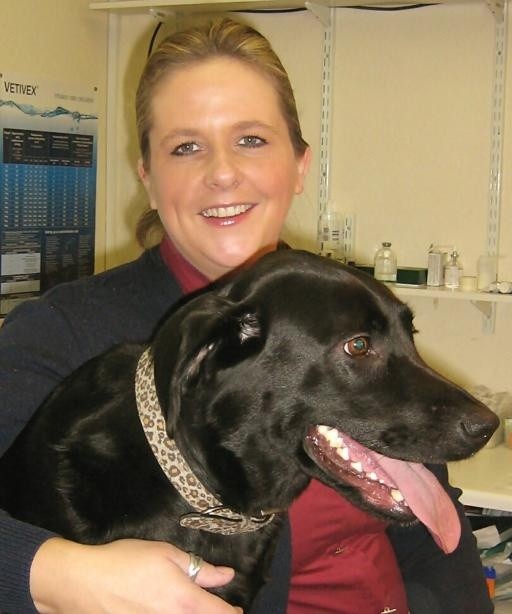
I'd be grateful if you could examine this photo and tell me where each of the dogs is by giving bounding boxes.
[0,250,500,614]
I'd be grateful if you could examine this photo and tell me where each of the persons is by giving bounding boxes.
[2,15,494,612]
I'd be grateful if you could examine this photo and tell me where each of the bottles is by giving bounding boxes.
[373,241,396,280]
[444,250,462,289]
[315,211,341,260]
[475,254,495,288]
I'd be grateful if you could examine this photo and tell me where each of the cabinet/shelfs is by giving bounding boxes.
[89,0,512,513]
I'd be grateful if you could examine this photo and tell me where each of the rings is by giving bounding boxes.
[185,549,204,582]
